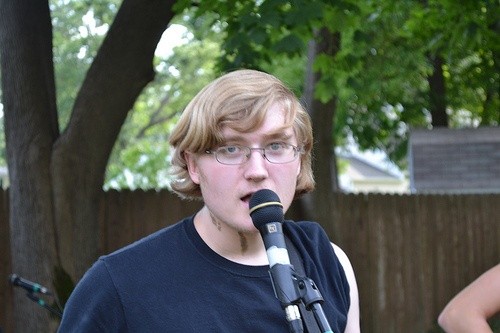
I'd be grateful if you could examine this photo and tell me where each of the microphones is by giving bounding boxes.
[9,274,51,295]
[249,189,304,333]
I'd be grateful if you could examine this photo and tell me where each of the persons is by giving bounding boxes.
[57,67,362,333]
[436,260,500,332]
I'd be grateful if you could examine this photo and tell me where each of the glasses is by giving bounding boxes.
[203,141,302,166]
